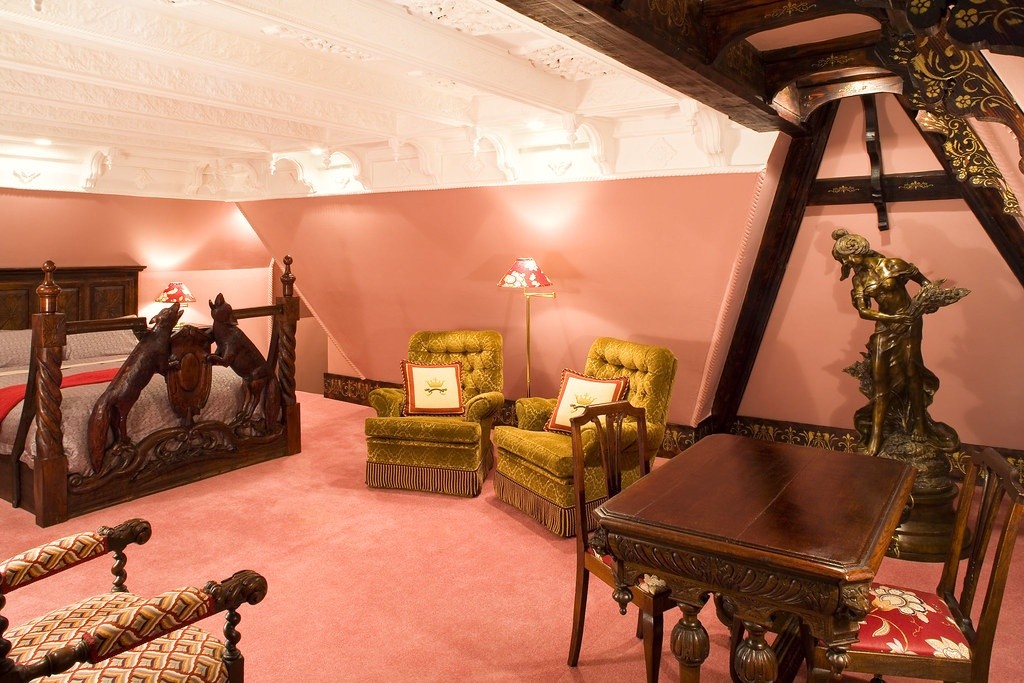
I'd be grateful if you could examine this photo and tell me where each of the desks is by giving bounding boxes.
[591,434,919,683]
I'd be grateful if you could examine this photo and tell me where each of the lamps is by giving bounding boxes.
[497,259,558,401]
[154,282,196,328]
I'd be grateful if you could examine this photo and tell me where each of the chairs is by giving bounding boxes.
[0,510,267,683]
[567,401,676,683]
[814,448,1024,683]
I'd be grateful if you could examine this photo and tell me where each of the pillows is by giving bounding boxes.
[399,357,464,417]
[544,368,628,438]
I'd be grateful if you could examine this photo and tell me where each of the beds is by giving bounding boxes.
[0,255,303,528]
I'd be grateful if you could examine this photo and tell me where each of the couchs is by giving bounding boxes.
[365,330,503,499]
[491,335,677,538]
